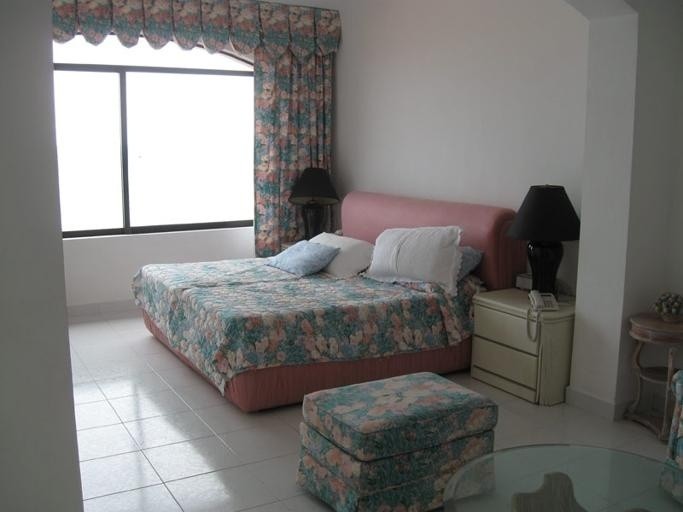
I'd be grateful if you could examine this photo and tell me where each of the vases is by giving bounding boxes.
[661,312,679,323]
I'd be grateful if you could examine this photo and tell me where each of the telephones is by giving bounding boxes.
[528,290,560,311]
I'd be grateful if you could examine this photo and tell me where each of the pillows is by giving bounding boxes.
[262,223,484,297]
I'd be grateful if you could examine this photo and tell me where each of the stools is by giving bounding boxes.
[625,313,681,443]
[297,371,500,511]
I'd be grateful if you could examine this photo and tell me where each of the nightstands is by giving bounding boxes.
[467,288,575,407]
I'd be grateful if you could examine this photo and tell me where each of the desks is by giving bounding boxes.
[443,443,682,510]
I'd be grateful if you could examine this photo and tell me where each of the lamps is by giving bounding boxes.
[286,167,340,241]
[503,183,580,299]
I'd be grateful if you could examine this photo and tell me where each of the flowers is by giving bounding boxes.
[652,291,682,314]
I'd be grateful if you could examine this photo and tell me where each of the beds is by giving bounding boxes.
[130,191,527,412]
